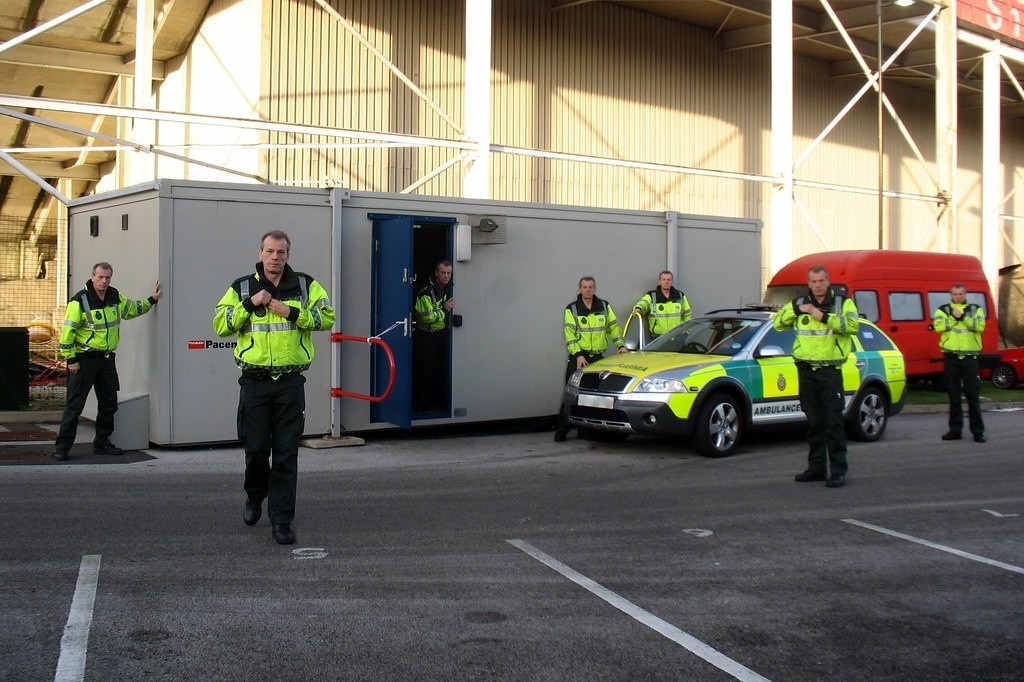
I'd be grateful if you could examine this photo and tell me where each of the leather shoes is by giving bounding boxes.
[973,434,986,442]
[942,431,962,440]
[795,470,826,482]
[825,475,846,488]
[272,525,295,544]
[94,446,123,455]
[54,447,69,461]
[243,498,262,525]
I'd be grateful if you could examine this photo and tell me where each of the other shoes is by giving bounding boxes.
[554,431,568,442]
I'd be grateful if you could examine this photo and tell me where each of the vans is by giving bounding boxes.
[764,252,1000,386]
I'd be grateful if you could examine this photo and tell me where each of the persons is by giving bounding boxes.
[212,230,336,545]
[773,266,859,487]
[554,277,627,442]
[413,260,456,411]
[934,284,987,443]
[53,262,162,461]
[632,271,694,343]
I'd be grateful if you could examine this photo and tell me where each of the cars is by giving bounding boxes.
[991,346,1024,390]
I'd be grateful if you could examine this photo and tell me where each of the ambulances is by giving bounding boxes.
[562,301,908,457]
